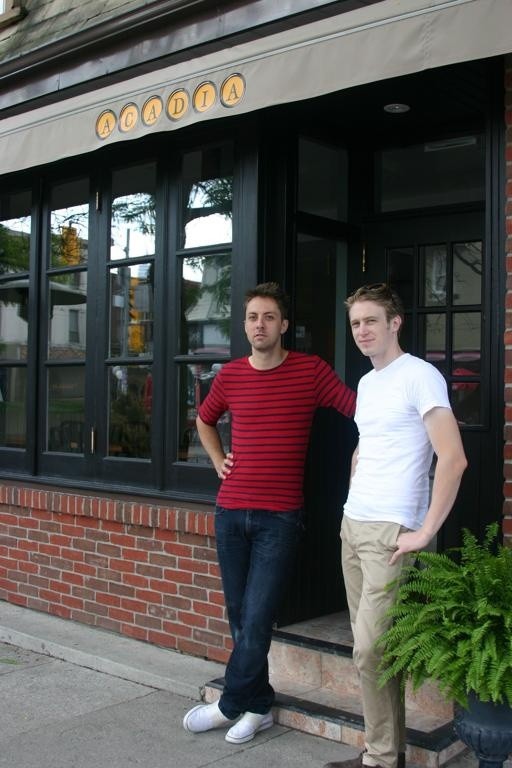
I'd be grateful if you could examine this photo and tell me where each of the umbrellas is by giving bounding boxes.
[323,752,381,767]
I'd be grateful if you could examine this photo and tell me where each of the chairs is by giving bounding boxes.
[224,708,274,744]
[183,700,233,734]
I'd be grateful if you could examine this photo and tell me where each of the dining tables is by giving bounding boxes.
[368,523,511,768]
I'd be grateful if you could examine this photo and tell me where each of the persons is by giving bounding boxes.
[180,280,356,745]
[324,282,470,766]
